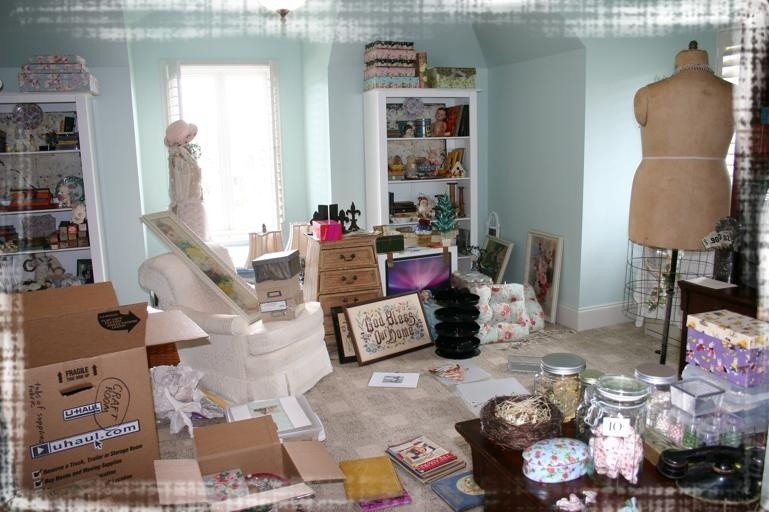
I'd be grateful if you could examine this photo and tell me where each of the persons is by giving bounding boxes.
[161,118,208,244]
[429,107,448,137]
[621,48,740,254]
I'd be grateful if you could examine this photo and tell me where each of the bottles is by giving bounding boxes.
[532,351,676,488]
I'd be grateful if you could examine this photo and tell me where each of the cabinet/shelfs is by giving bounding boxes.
[1,92,107,291]
[302,236,384,342]
[366,83,477,250]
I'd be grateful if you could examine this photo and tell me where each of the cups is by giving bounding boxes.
[671,378,725,415]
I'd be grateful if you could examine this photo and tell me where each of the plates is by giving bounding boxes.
[389,164,405,180]
[12,104,43,130]
[55,177,90,207]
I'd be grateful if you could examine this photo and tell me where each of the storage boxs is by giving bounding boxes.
[154,413,347,512]
[0,281,210,512]
[682,363,767,435]
[682,311,768,388]
[258,290,305,321]
[249,248,302,303]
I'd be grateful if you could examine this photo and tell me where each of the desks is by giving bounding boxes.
[453,417,767,511]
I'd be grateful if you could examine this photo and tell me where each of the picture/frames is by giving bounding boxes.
[478,236,515,283]
[141,212,258,318]
[523,231,564,326]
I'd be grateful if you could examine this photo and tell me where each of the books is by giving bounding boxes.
[336,452,404,504]
[356,484,416,510]
[428,470,487,511]
[446,147,465,173]
[385,432,467,487]
[447,105,471,137]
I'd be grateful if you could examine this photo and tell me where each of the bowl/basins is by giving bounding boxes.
[396,119,432,137]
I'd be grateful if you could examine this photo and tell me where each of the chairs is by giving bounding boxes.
[139,239,333,405]
